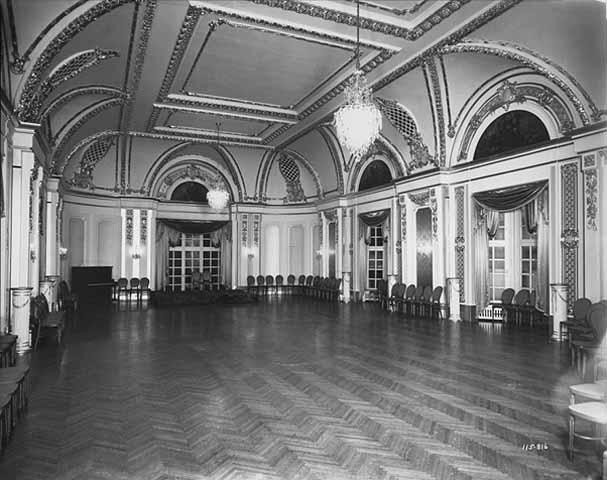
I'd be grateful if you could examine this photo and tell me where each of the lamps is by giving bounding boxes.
[206,121,231,211]
[331,0,383,162]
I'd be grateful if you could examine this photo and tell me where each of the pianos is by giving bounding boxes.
[71,267,115,307]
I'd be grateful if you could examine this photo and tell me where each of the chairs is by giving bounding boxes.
[34,281,79,353]
[492,288,536,329]
[247,274,341,303]
[118,278,151,302]
[378,279,444,320]
[0,336,31,450]
[559,298,607,480]
